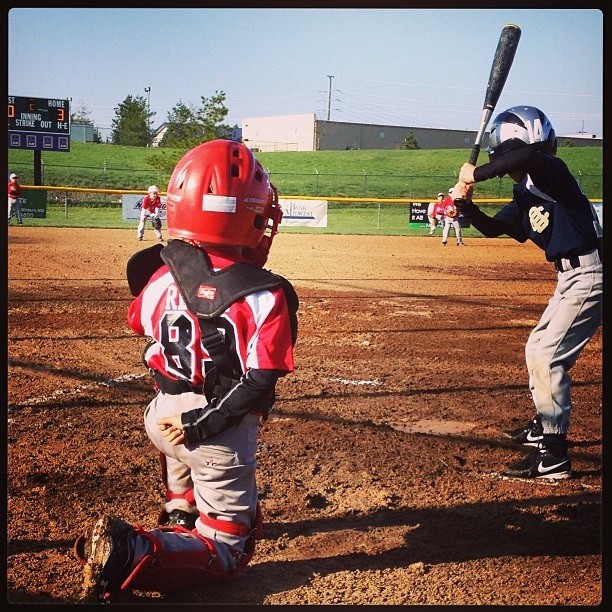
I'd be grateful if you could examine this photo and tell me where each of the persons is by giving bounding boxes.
[138,186,166,242]
[442,188,465,246]
[428,192,445,237]
[8,173,23,226]
[80,139,298,606]
[450,105,602,482]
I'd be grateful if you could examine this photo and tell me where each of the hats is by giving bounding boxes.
[10,174,20,179]
[448,188,454,193]
[437,193,444,197]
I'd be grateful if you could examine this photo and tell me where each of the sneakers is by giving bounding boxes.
[442,242,447,245]
[81,514,138,605]
[139,238,143,240]
[458,242,465,246]
[503,440,574,480]
[500,412,548,452]
[158,238,163,241]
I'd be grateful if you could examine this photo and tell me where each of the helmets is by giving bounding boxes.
[148,185,160,194]
[165,139,284,268]
[484,106,558,179]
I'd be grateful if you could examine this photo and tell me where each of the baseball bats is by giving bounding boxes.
[454,24,521,208]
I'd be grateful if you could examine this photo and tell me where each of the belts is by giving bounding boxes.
[553,257,580,273]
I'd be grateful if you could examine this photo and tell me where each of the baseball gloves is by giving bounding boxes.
[448,209,458,217]
[432,218,438,226]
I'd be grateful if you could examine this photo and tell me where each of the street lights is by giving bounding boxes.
[144,86,150,146]
